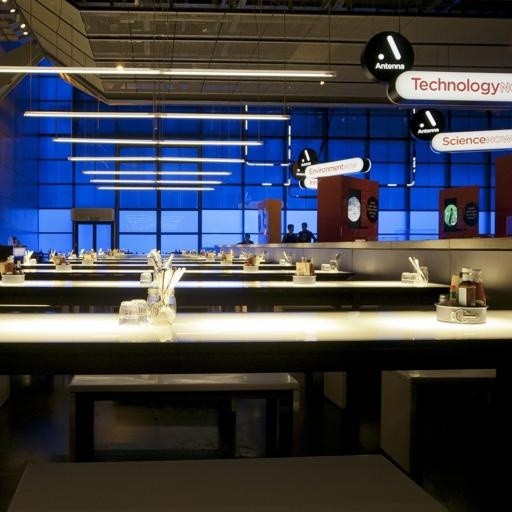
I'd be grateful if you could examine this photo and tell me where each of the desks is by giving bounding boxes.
[0,253,512,512]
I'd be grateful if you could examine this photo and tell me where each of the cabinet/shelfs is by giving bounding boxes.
[257,175,479,243]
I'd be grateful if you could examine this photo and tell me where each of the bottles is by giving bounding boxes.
[437,266,487,307]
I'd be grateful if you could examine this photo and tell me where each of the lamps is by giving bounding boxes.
[1,0,340,91]
[63,150,247,195]
[17,73,297,127]
[43,119,274,151]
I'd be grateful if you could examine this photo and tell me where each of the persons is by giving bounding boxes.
[295,222,317,243]
[242,233,254,244]
[279,223,298,243]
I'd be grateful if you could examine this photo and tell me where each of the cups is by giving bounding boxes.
[118,298,149,327]
